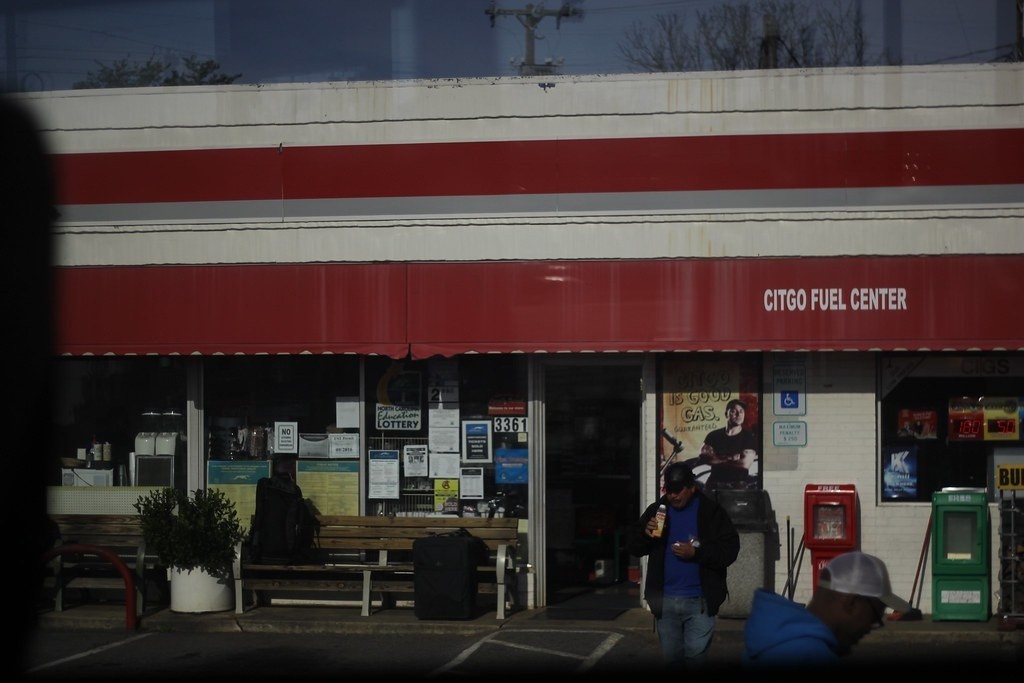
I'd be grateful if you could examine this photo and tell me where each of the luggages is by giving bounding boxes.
[411,534,477,620]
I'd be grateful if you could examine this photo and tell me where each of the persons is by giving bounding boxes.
[627,462,741,664]
[700,400,754,490]
[741,553,911,661]
[239,460,302,566]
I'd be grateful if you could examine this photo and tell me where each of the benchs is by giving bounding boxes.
[232,514,520,620]
[43,514,168,615]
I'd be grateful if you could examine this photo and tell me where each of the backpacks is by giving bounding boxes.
[249,473,304,560]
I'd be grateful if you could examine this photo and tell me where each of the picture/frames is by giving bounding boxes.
[462,420,492,463]
[368,449,399,499]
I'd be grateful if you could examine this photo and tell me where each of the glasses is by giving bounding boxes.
[666,487,682,494]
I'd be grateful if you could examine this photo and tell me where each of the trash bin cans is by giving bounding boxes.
[707,488,773,620]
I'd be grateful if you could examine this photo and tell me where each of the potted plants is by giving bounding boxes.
[129,487,248,613]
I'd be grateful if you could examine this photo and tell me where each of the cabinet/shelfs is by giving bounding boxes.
[931,487,989,621]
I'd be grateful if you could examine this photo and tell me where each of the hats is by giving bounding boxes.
[727,399,748,409]
[663,463,695,486]
[817,552,912,615]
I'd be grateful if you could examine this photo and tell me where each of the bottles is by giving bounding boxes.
[90,435,102,461]
[651,505,665,537]
[103,442,112,461]
